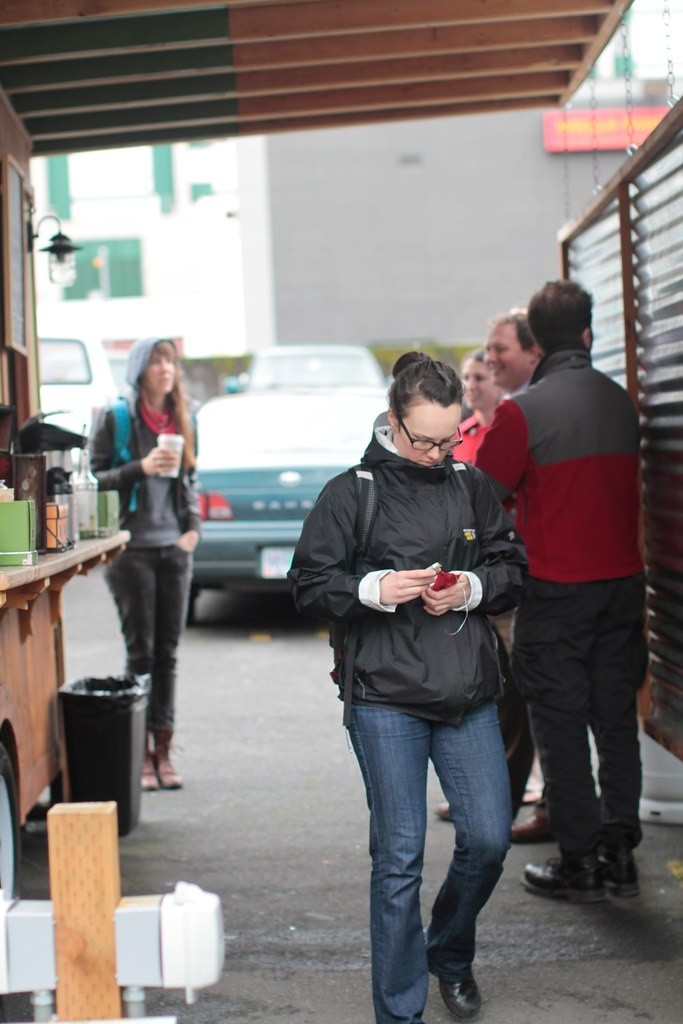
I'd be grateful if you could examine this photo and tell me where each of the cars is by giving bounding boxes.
[224,346,394,395]
[186,387,391,625]
[38,327,117,472]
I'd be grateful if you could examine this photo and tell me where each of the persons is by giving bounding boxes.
[91,337,201,789]
[435,310,555,844]
[476,280,646,903]
[286,351,530,1024]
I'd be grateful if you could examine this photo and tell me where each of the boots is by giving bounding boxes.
[154,727,183,789]
[140,730,159,792]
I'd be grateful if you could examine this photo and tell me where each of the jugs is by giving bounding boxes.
[19,423,89,470]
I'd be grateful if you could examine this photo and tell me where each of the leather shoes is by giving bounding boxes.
[426,953,481,1018]
[599,855,639,898]
[511,815,554,845]
[435,803,451,822]
[520,854,608,902]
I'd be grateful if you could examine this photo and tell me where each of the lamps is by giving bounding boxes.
[26,214,83,285]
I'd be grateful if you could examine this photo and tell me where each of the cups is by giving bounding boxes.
[156,434,184,478]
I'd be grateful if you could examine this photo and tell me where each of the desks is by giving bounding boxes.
[0,529,130,643]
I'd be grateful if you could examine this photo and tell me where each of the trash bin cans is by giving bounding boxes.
[56,674,152,837]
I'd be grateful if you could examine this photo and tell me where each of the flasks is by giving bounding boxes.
[46,467,74,544]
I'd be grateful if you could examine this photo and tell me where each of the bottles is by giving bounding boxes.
[71,449,99,539]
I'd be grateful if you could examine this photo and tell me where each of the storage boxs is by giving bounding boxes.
[0,491,119,566]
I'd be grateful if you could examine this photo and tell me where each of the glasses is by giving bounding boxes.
[396,412,462,450]
